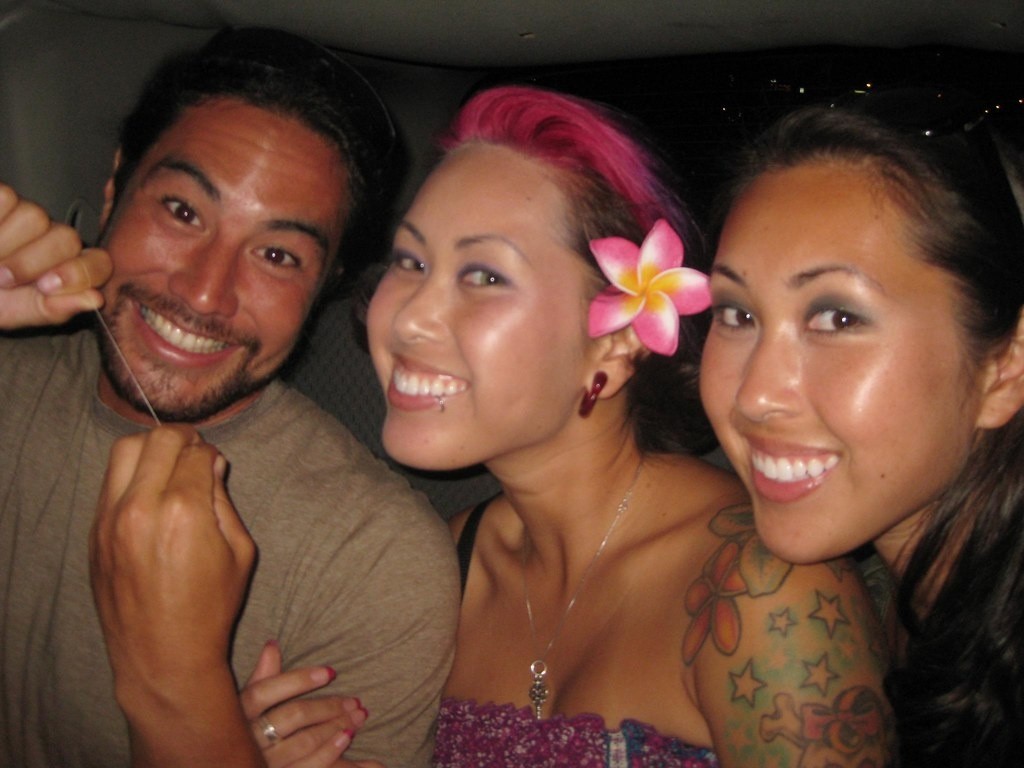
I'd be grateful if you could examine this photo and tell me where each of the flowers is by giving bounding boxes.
[587,218,712,356]
[681,501,854,667]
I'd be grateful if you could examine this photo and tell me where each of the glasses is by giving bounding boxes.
[719,66,1024,137]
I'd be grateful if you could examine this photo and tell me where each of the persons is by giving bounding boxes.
[232,88,897,767]
[0,25,461,767]
[693,101,1024,768]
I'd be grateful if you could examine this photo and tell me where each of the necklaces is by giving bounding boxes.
[521,448,645,722]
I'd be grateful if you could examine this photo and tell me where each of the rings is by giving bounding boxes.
[257,713,283,746]
